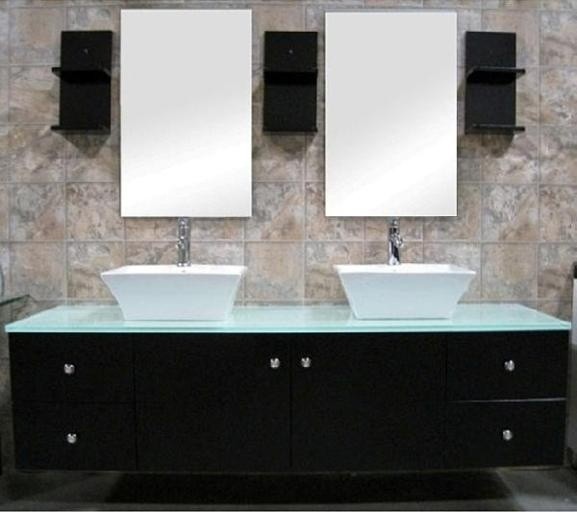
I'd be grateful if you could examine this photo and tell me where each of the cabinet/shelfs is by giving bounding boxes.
[260,30,319,133]
[463,30,525,135]
[8,333,568,470]
[50,30,113,135]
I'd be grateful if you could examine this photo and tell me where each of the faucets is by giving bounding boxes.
[175,218,190,265]
[387,217,402,266]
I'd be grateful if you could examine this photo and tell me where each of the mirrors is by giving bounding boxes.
[324,12,458,219]
[120,9,252,220]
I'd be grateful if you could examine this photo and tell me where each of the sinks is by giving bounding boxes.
[334,264,477,320]
[101,265,242,320]
